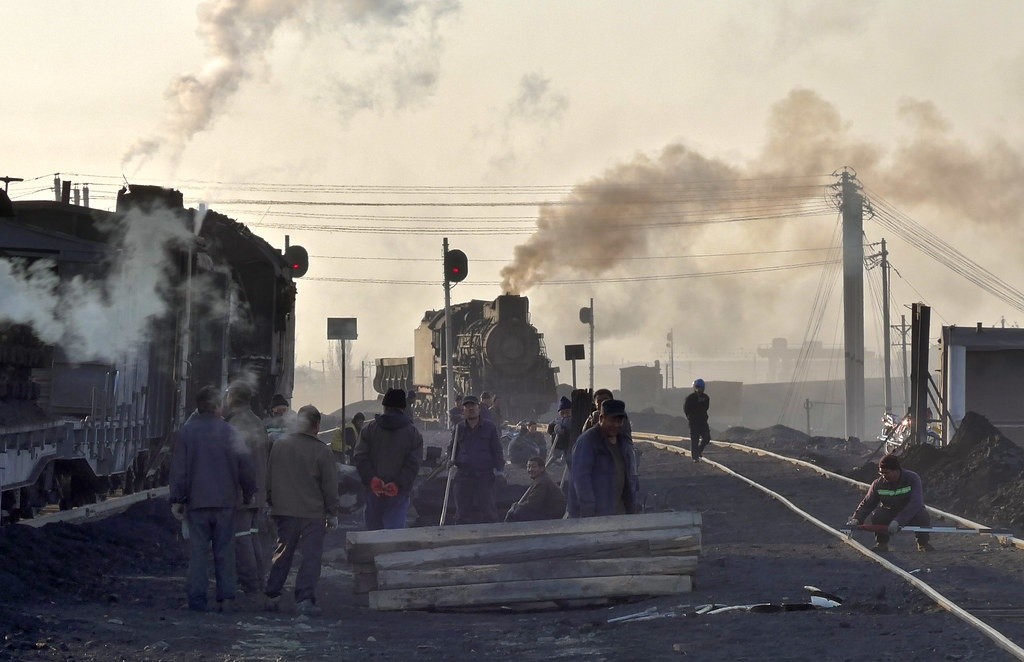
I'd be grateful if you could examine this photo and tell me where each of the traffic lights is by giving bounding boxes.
[446,250,468,283]
[285,245,308,278]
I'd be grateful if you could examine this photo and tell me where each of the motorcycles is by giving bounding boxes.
[876,407,941,456]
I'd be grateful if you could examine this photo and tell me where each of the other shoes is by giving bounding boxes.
[191,602,233,613]
[248,579,268,592]
[693,446,702,463]
[267,596,322,616]
[871,542,935,552]
[177,604,189,610]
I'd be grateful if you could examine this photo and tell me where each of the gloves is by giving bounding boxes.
[845,519,899,534]
[370,479,398,497]
[327,518,338,529]
[249,495,256,504]
[266,506,272,519]
[554,424,561,433]
[171,503,186,520]
[447,460,456,469]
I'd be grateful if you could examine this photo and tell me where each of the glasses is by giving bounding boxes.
[878,472,889,476]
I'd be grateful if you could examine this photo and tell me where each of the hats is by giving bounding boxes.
[462,396,479,405]
[558,396,572,412]
[879,455,901,470]
[480,392,491,398]
[382,388,406,409]
[408,391,416,397]
[601,399,627,418]
[455,396,462,401]
[225,381,250,403]
[270,394,289,408]
[520,425,529,435]
[354,413,365,421]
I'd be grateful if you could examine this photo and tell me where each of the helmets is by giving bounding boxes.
[692,378,705,387]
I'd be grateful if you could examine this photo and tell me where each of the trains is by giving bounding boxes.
[372,294,559,424]
[0,179,296,524]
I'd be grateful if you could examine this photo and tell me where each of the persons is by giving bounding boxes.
[169,387,258,616]
[354,388,423,529]
[403,390,416,422]
[685,378,711,463]
[447,386,638,526]
[330,411,364,466]
[847,455,933,553]
[225,380,270,601]
[264,394,297,450]
[262,405,340,617]
